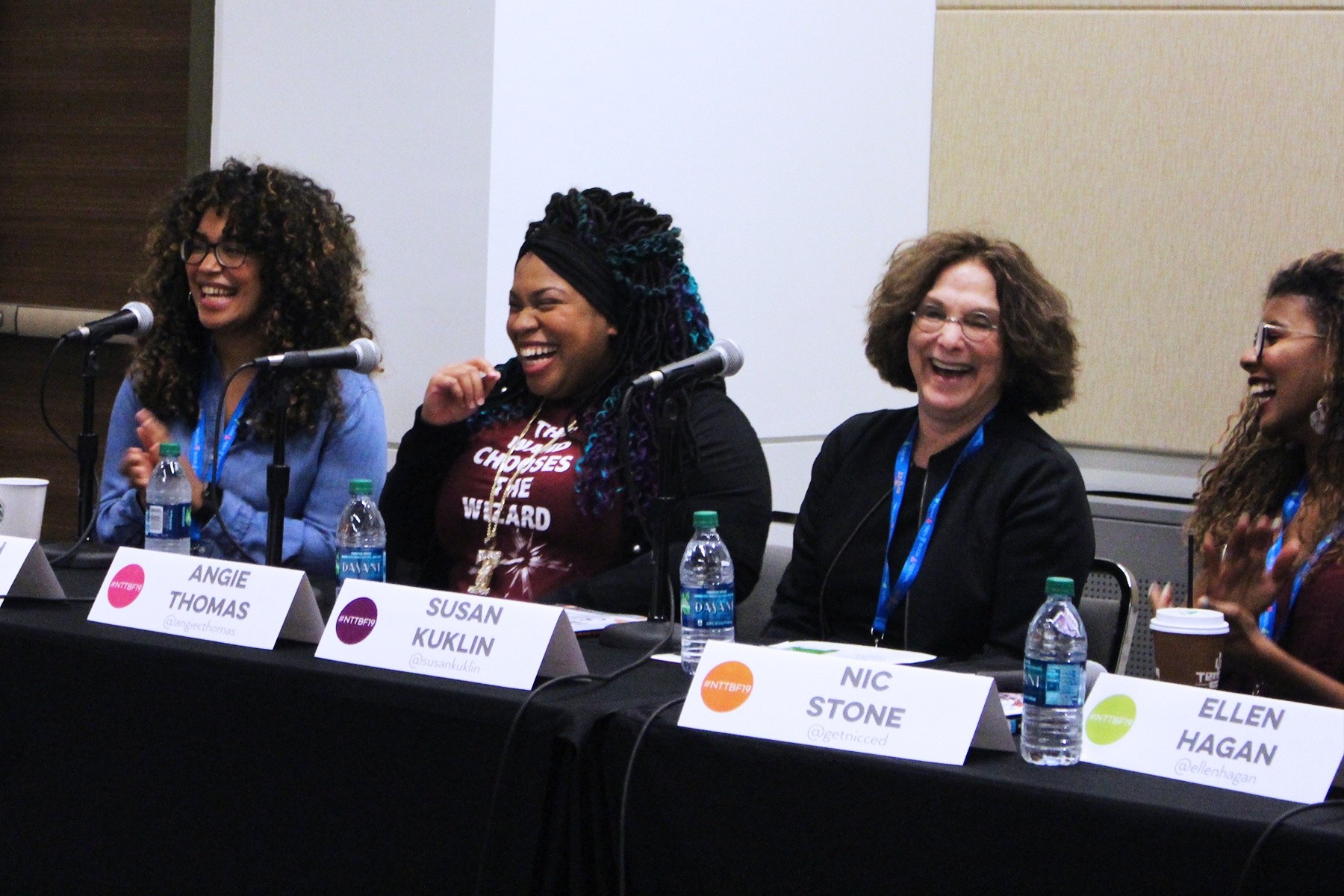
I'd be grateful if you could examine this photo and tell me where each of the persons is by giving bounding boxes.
[1145,248,1344,721]
[761,231,1097,663]
[93,163,391,586]
[374,187,772,631]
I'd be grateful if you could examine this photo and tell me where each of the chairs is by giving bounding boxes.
[736,509,800,646]
[1078,558,1139,676]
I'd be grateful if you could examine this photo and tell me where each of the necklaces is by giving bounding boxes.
[467,402,582,597]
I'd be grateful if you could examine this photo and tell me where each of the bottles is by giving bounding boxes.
[336,479,387,602]
[145,443,192,558]
[680,511,735,676]
[1020,577,1089,767]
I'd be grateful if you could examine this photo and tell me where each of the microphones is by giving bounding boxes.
[251,338,383,374]
[61,302,154,341]
[632,338,745,392]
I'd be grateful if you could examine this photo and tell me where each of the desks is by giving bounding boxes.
[0,554,1344,896]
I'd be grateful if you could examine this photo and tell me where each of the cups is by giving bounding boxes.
[0,477,50,544]
[1149,607,1231,689]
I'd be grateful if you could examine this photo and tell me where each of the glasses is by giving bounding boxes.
[910,306,1005,343]
[1253,320,1325,361]
[182,233,264,268]
